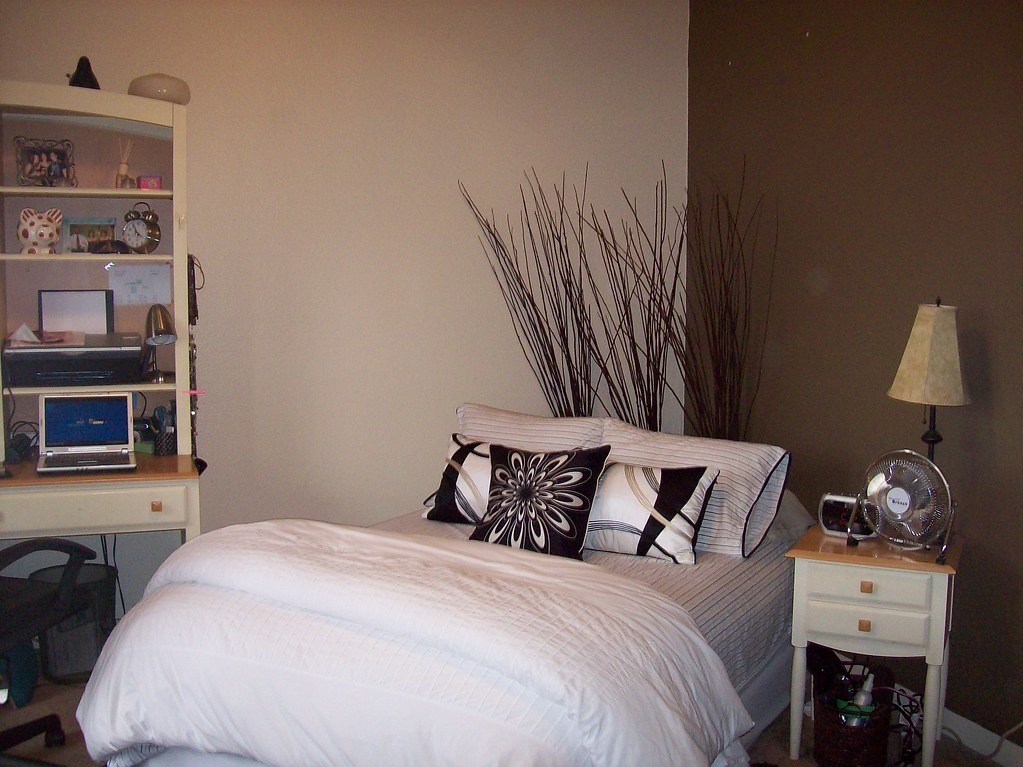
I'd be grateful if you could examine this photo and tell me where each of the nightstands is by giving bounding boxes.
[786,530,960,767]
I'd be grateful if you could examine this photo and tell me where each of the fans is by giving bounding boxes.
[848,447,958,564]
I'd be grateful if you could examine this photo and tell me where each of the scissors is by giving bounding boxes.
[153,406,168,433]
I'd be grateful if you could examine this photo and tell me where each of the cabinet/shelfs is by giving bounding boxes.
[0,76,199,542]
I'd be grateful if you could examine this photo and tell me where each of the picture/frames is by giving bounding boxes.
[61,216,117,255]
[14,136,79,188]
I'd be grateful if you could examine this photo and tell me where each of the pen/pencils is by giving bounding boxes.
[149,424,156,434]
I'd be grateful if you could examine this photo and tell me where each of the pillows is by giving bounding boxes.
[469,445,611,562]
[456,405,601,451]
[602,416,791,558]
[421,432,497,526]
[584,461,721,565]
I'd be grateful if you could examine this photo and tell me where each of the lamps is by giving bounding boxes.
[144,304,179,381]
[884,294,972,543]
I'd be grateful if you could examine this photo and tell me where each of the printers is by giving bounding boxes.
[3,332,143,388]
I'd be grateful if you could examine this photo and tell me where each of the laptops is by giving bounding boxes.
[36,392,137,473]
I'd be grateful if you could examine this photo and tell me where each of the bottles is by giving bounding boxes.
[845,673,874,727]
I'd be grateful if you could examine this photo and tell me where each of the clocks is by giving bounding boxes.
[121,202,161,255]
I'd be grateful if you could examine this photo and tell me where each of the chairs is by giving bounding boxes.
[0,537,98,767]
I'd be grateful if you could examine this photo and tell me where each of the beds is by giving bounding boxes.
[76,404,819,765]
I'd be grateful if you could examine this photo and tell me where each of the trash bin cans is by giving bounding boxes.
[812,660,894,767]
[27,563,119,684]
